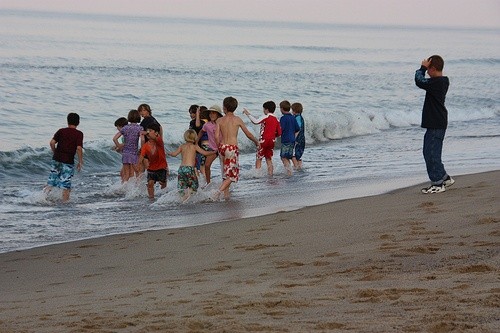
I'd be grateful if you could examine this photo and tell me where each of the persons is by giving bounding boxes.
[188,104,207,175]
[168,129,217,201]
[215,96,260,203]
[138,124,168,199]
[45,113,84,203]
[291,102,306,169]
[242,101,282,180]
[279,100,300,178]
[415,54,456,194]
[138,104,162,170]
[114,110,145,183]
[188,104,223,189]
[114,117,129,131]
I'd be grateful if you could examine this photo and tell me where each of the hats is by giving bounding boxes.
[206,104,223,117]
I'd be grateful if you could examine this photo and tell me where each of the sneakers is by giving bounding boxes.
[443,175,455,187]
[421,182,445,194]
